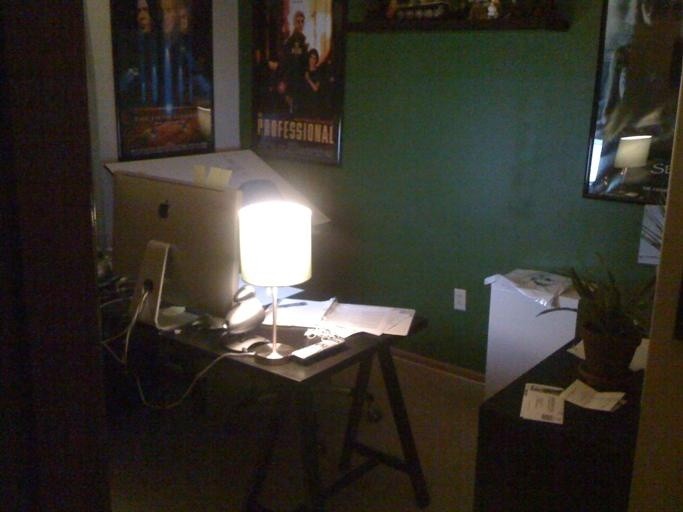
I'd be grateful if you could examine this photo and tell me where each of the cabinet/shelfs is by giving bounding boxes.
[475,331,648,511]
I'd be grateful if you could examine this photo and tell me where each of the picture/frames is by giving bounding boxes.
[254,2,344,164]
[362,0,573,30]
[108,0,213,161]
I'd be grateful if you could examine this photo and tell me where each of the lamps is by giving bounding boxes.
[613,133,652,197]
[238,201,312,363]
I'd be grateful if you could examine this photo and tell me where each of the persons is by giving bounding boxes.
[600,0,681,161]
[252,9,329,116]
[117,1,212,100]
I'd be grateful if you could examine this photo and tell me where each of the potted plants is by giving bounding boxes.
[533,250,656,383]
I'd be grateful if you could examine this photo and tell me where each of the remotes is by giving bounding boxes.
[291,339,346,364]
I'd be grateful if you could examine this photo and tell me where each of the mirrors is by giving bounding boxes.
[583,0,683,207]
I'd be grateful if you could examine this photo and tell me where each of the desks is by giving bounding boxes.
[101,286,429,512]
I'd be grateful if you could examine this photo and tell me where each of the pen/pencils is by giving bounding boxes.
[281,303,308,309]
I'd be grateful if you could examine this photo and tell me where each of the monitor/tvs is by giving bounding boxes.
[589,138,603,186]
[111,170,243,332]
